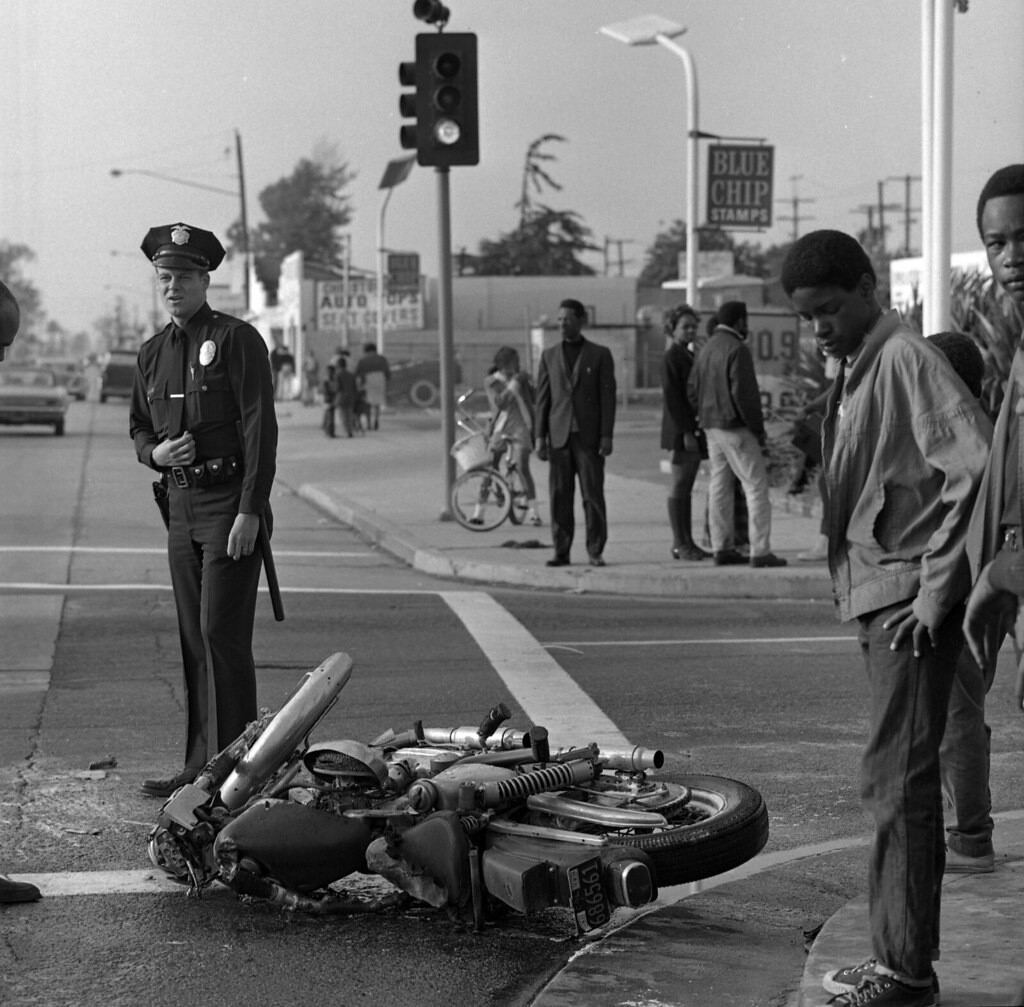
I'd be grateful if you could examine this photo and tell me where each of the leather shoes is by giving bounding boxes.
[139,766,201,797]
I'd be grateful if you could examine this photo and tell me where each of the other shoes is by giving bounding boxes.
[748,551,788,568]
[0,872,40,903]
[547,554,570,567]
[798,541,830,560]
[714,549,751,565]
[588,552,607,567]
[943,844,998,872]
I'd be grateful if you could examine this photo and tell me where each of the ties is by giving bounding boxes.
[168,325,186,443]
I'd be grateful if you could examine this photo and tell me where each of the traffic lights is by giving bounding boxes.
[400,31,482,167]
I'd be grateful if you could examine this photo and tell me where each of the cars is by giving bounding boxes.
[0,364,69,435]
[50,359,90,400]
[98,348,140,402]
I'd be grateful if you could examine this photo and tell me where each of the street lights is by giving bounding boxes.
[378,158,416,353]
[111,167,249,322]
[602,11,700,312]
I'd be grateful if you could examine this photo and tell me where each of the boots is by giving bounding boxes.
[665,493,714,561]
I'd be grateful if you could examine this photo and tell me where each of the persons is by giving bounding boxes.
[660,303,714,560]
[685,302,787,569]
[964,164,1024,712]
[279,344,297,399]
[303,349,319,406]
[0,284,43,904]
[332,357,364,437]
[356,343,390,429]
[703,317,750,551]
[270,341,281,398]
[321,364,335,437]
[780,227,994,1007]
[129,222,278,798]
[536,301,617,567]
[469,345,541,526]
[925,332,993,872]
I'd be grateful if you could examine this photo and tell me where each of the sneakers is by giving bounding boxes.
[822,953,941,1007]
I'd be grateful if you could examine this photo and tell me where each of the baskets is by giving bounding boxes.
[450,432,493,472]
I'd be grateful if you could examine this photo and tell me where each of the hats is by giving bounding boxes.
[141,223,225,275]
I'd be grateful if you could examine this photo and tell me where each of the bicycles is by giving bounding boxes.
[452,388,529,531]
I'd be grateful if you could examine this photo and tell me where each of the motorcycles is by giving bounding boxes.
[148,651,769,945]
